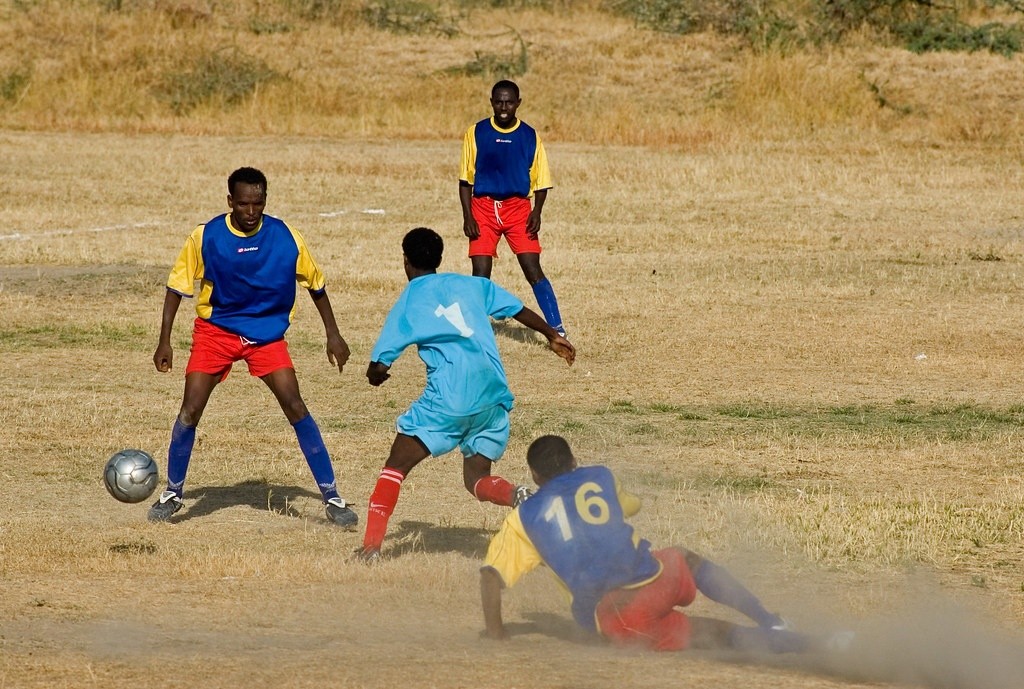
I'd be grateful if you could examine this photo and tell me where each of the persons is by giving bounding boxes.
[147,167,359,527]
[352,227,576,563]
[478,436,857,656]
[458,80,568,340]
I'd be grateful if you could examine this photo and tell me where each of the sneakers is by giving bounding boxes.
[347,547,385,571]
[511,485,539,509]
[145,490,185,526]
[322,497,360,527]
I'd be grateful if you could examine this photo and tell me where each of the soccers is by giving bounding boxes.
[105,450,158,502]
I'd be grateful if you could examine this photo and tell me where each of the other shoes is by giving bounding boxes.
[767,615,809,651]
[552,324,566,338]
